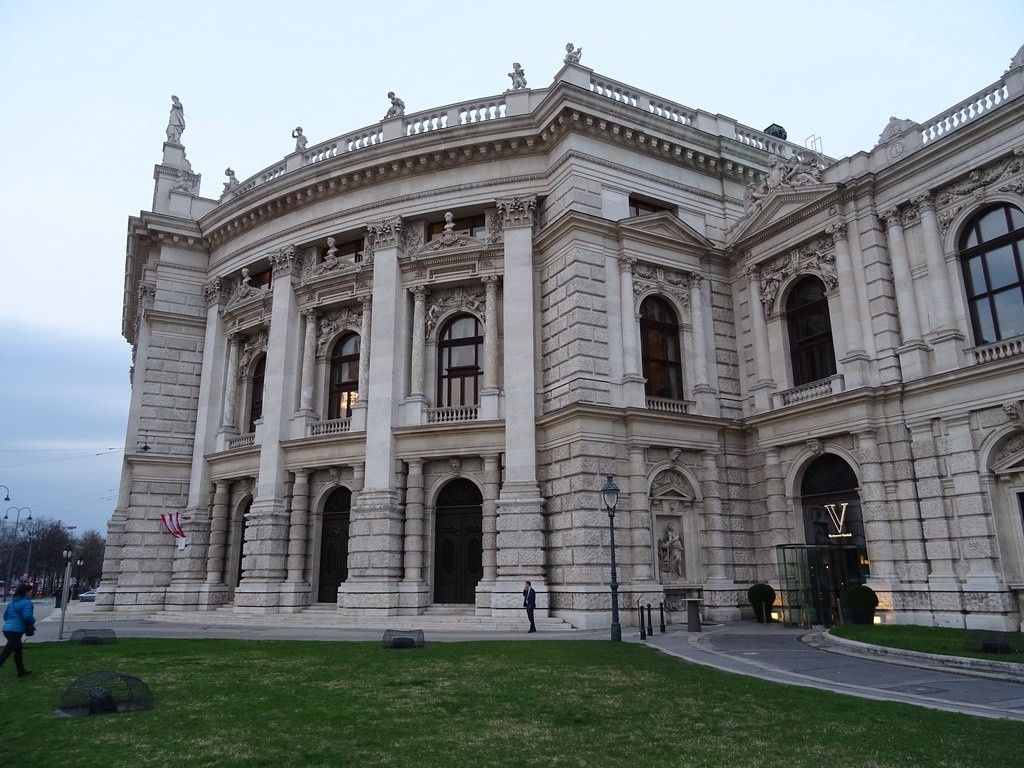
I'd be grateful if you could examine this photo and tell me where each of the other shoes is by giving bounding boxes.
[18,669,31,677]
[528,628,536,633]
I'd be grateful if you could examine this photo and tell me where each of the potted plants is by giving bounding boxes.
[843,585,879,625]
[748,583,776,623]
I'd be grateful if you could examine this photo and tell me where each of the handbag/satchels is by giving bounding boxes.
[26,621,34,638]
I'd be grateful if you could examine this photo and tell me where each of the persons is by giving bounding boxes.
[743,155,821,217]
[759,251,839,317]
[672,448,681,464]
[806,439,824,454]
[444,212,456,231]
[563,43,582,63]
[315,314,363,357]
[384,92,405,118]
[0,584,36,677]
[327,237,339,255]
[292,127,308,152]
[329,466,340,483]
[240,343,253,368]
[241,479,253,493]
[222,167,239,193]
[425,296,485,340]
[969,170,983,183]
[523,581,536,633]
[173,169,190,191]
[449,458,462,475]
[1002,402,1024,427]
[166,95,185,145]
[242,268,252,284]
[665,523,683,577]
[54,584,92,608]
[508,63,526,89]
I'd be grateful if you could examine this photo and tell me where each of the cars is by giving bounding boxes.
[78,590,97,602]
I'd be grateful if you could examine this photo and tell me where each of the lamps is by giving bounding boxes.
[393,638,416,648]
[82,637,103,645]
[89,687,118,715]
[142,445,151,450]
[982,639,1013,654]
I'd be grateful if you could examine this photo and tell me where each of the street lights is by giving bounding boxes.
[75,556,84,586]
[600,472,622,642]
[2,506,32,602]
[57,543,72,590]
